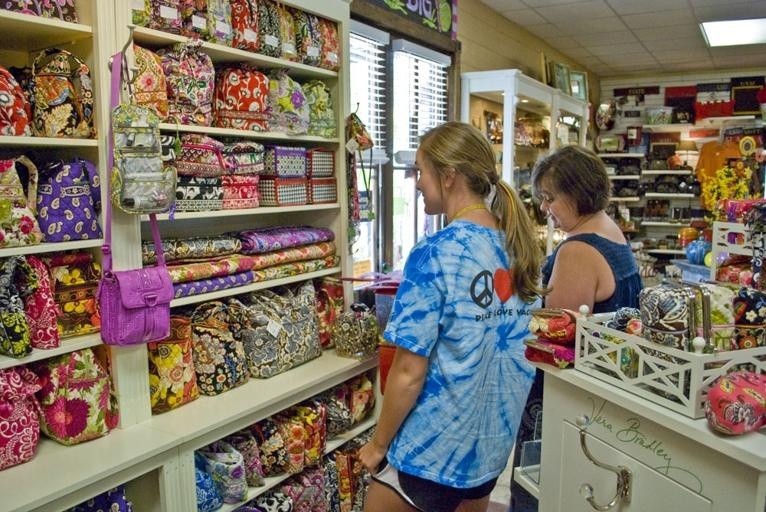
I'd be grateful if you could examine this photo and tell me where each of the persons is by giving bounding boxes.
[352,122,553,512]
[509,146,643,512]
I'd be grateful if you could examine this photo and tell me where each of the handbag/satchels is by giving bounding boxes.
[314,278,345,353]
[38,348,119,448]
[247,277,322,378]
[149,316,199,414]
[184,301,249,396]
[0,363,44,470]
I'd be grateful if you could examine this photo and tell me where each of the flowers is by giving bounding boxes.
[697,163,761,230]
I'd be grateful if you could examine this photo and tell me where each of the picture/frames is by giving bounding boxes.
[552,61,573,96]
[541,53,551,84]
[570,70,589,101]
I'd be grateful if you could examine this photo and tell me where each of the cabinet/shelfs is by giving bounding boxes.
[0,0,381,511]
[461,69,592,256]
[596,81,765,277]
[514,355,766,512]
